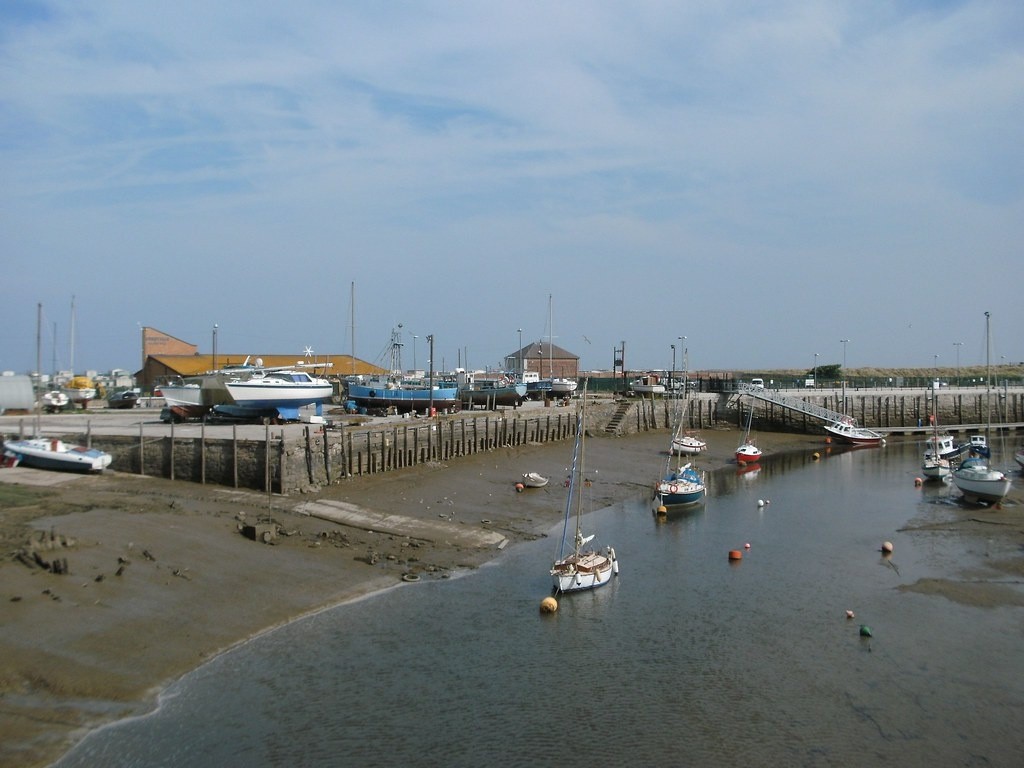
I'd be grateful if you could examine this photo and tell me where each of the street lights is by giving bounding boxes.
[841,339,850,386]
[952,343,964,387]
[814,353,820,389]
[934,354,939,381]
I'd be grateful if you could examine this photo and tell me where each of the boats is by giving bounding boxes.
[921,434,990,481]
[824,414,890,446]
[630,377,700,393]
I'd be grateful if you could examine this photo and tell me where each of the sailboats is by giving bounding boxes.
[657,344,706,513]
[667,336,706,455]
[5,302,115,471]
[0,282,577,425]
[552,377,614,595]
[950,310,1015,500]
[735,383,763,465]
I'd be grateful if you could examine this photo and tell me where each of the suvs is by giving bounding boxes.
[751,378,764,390]
[805,374,814,388]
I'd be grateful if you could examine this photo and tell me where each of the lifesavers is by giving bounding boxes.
[656,482,659,491]
[670,485,678,494]
